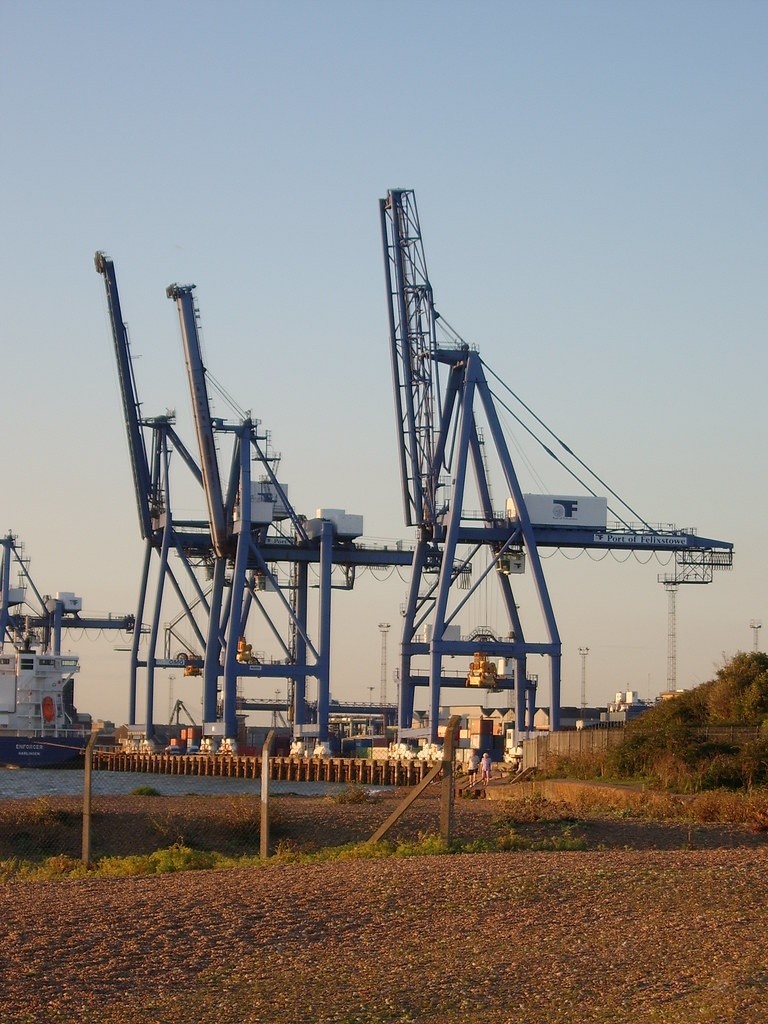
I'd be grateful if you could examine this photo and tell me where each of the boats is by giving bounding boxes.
[0,615,97,770]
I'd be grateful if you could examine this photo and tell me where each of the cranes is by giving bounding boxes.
[0,529,152,655]
[379,186,736,760]
[94,250,474,757]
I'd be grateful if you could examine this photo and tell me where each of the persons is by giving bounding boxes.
[466,750,480,788]
[480,753,492,786]
